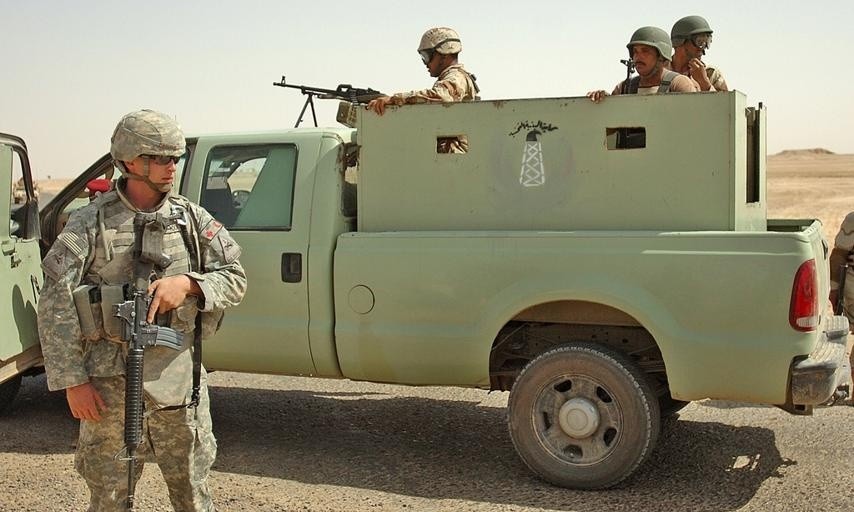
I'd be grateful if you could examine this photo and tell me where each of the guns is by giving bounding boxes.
[111,212,184,508]
[273,75,399,127]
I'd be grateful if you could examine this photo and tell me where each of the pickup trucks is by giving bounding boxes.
[0,90,847,493]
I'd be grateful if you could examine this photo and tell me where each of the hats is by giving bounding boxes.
[417,27,462,56]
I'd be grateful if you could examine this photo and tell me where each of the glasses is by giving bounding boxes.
[138,154,181,165]
[690,32,712,49]
[419,49,435,65]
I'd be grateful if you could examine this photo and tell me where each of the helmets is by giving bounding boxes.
[109,108,187,164]
[670,15,713,48]
[626,26,673,62]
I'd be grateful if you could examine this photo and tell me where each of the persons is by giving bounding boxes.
[662,15,729,91]
[829,211,854,408]
[13,174,40,206]
[37,109,247,511]
[364,28,480,153]
[586,26,697,147]
